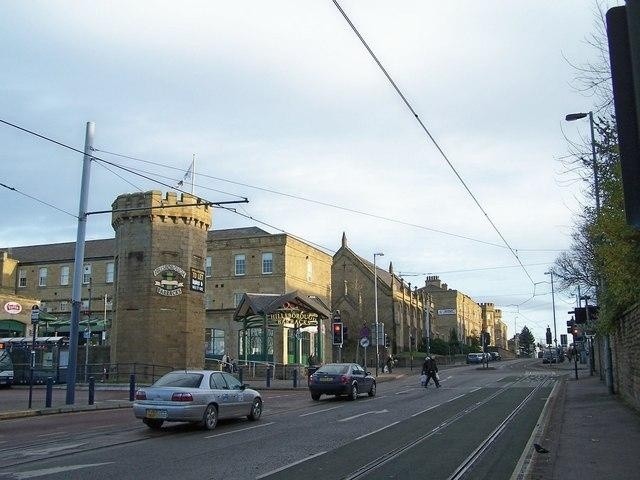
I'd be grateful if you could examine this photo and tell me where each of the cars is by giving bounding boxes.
[308,362,376,400]
[466,352,501,363]
[132,367,264,434]
[538,347,568,363]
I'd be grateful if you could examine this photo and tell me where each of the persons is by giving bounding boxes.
[420,353,442,388]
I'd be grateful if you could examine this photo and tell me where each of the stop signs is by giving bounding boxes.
[333,314,341,322]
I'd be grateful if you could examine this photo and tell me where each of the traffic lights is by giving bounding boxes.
[331,322,343,345]
[567,321,582,338]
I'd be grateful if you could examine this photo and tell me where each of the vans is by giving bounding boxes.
[0,349,14,388]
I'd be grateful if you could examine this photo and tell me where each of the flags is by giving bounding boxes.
[175,160,193,190]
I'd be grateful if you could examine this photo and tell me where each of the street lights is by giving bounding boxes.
[374,252,385,377]
[544,271,559,364]
